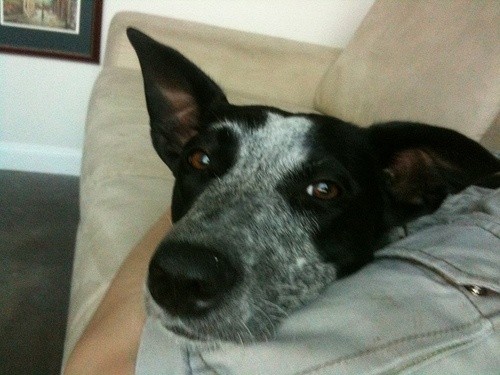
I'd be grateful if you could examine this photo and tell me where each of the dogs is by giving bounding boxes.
[126,26,500,346]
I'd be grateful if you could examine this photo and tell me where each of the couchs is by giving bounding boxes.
[62,0,500,375]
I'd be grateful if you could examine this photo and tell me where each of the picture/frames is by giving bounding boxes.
[0,0,103,66]
